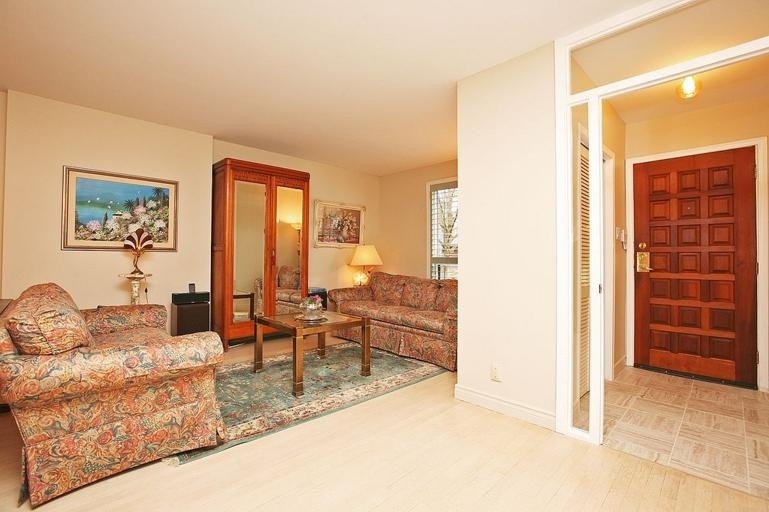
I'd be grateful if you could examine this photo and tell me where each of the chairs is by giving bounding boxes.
[0,301,224,510]
[254,264,301,313]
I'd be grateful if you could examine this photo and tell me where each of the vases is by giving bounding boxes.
[300,303,323,316]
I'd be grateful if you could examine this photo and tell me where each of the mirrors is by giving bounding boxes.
[233,180,264,323]
[276,185,303,314]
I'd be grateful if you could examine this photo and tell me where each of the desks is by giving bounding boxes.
[119,274,152,305]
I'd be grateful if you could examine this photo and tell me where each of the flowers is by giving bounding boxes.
[304,295,324,305]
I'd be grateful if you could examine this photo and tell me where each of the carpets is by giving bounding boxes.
[161,342,448,467]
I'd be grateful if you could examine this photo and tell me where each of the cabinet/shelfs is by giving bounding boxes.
[211,158,308,353]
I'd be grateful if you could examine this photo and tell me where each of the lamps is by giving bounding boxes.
[290,223,300,265]
[123,228,153,275]
[350,246,383,286]
[675,77,702,99]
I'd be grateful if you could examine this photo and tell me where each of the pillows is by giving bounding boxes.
[4,283,95,356]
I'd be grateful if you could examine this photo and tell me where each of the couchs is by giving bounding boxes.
[328,272,457,372]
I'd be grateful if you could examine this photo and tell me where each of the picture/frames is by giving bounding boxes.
[61,165,179,252]
[313,200,366,249]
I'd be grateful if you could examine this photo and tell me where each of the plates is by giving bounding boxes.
[297,319,329,325]
[294,314,326,321]
